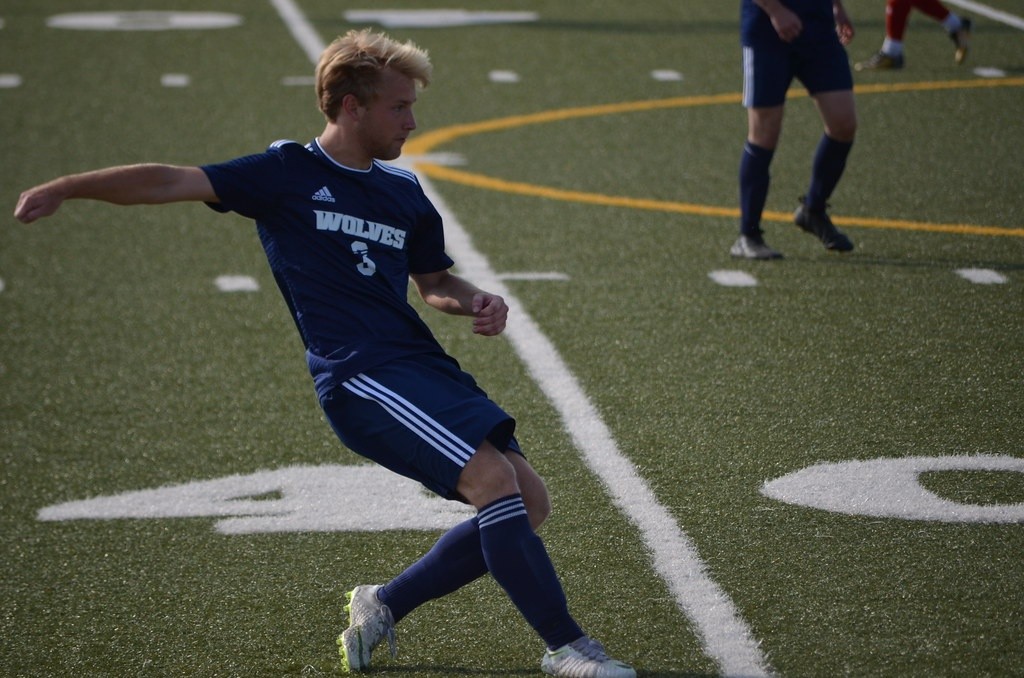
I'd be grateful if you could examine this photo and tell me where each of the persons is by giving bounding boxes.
[13,27,634,678]
[728,1,857,261]
[850,0,974,74]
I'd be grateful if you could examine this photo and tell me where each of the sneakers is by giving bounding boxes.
[731,239,781,259]
[541,635,638,678]
[854,50,905,70]
[950,18,972,64]
[335,584,397,673]
[794,199,854,251]
